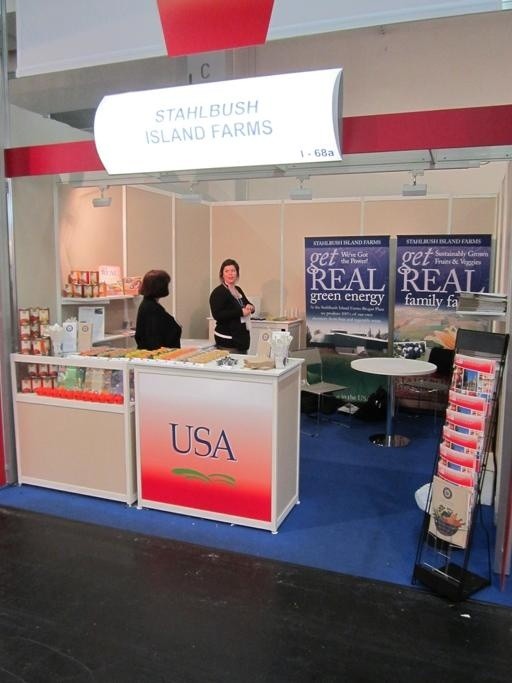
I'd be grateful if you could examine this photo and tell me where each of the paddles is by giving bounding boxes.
[1,402,511,605]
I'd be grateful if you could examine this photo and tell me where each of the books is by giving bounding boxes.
[477,451,495,506]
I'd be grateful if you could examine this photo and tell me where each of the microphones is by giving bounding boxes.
[415,482,463,550]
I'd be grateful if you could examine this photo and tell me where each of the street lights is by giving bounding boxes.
[360,394,387,421]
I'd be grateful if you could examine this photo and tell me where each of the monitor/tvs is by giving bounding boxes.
[429,354,501,548]
[455,290,506,312]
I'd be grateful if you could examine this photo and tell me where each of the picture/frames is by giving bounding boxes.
[180,339,217,351]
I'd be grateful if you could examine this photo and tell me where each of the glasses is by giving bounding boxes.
[92,186,112,207]
[402,170,427,195]
[182,182,201,203]
[290,175,312,200]
[98,266,121,295]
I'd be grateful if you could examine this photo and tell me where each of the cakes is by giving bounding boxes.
[18,307,52,356]
[63,271,107,298]
[20,362,60,394]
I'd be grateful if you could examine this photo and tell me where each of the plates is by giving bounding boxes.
[10,352,138,507]
[207,317,306,358]
[62,295,143,348]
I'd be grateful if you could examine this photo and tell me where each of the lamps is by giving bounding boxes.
[393,341,450,433]
[288,348,351,437]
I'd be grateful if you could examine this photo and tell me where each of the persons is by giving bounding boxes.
[135,271,181,347]
[209,259,255,355]
